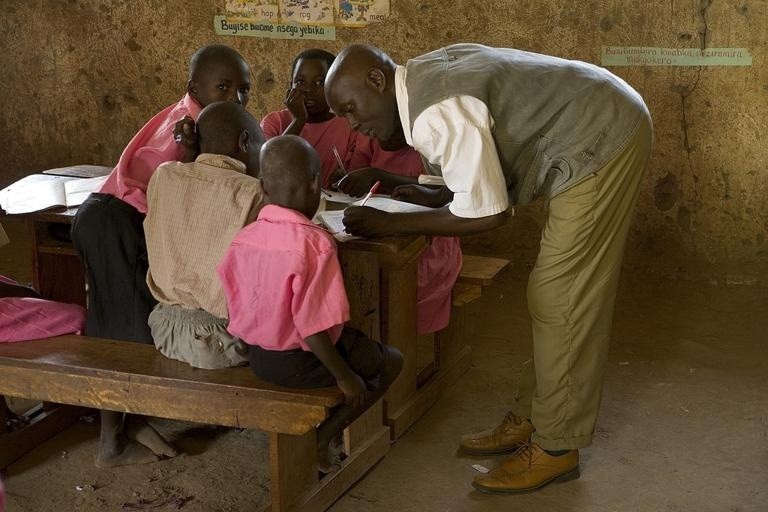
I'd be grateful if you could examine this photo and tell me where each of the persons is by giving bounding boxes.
[136,101,266,459]
[70,44,252,468]
[325,132,463,335]
[217,134,405,473]
[324,43,654,495]
[259,48,350,188]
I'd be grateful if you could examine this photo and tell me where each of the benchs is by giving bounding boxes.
[417,253,511,393]
[0,334,346,511]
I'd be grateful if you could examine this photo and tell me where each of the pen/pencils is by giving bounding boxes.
[332,143,347,175]
[320,187,333,198]
[343,180,381,232]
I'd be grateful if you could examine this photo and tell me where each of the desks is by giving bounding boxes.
[24,162,444,491]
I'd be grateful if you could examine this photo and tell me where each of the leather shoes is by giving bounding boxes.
[459,410,534,454]
[471,440,581,494]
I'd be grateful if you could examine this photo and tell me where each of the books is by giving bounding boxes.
[3,174,110,214]
